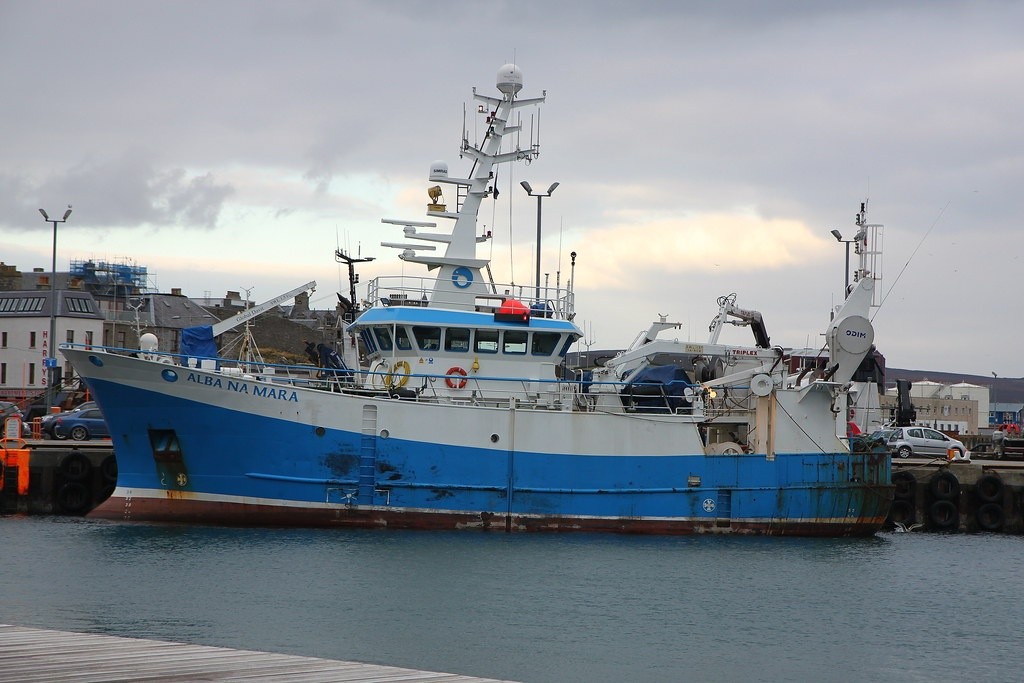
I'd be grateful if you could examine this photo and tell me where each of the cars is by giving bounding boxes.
[869,430,901,444]
[56,408,110,441]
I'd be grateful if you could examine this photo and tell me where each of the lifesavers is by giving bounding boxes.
[56,452,93,514]
[891,471,918,500]
[1007,424,1020,434]
[976,475,1004,503]
[386,360,410,388]
[887,500,915,530]
[100,454,118,483]
[929,471,960,500]
[976,503,1005,530]
[930,501,958,528]
[446,366,468,388]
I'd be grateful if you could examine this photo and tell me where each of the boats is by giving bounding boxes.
[58,62,900,537]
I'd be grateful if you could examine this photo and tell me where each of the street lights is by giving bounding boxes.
[993,372,998,431]
[41,209,71,407]
[519,180,561,305]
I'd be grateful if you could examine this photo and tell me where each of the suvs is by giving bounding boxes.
[847,422,860,437]
[886,427,968,459]
[40,401,99,440]
[0,401,24,438]
